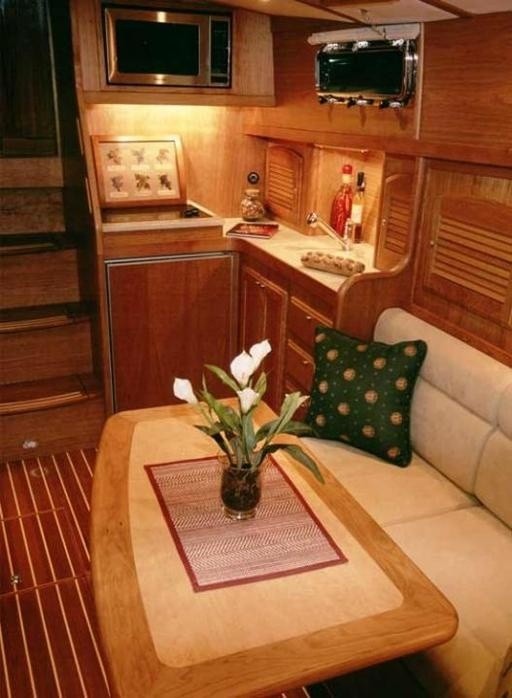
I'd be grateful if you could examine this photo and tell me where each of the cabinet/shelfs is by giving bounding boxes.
[104,252,238,414]
[0,190,104,463]
[238,253,338,423]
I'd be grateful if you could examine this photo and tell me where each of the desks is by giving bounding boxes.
[90,396,458,697]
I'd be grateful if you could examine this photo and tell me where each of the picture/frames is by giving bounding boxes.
[91,135,187,209]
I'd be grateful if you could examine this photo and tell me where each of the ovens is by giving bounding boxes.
[313,40,416,109]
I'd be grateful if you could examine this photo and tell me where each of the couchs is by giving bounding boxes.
[297,306,510,698]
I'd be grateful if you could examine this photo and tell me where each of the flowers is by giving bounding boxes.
[173,339,326,497]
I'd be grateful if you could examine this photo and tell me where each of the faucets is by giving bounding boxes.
[306,212,356,252]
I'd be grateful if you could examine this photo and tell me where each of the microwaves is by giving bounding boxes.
[101,6,232,89]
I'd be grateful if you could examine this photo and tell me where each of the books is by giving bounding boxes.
[226,221,280,241]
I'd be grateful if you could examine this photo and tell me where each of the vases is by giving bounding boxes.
[216,443,271,522]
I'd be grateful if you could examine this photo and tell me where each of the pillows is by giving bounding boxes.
[295,326,428,468]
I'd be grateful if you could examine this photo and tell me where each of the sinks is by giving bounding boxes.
[270,241,372,272]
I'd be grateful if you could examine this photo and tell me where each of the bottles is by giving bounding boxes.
[330,164,354,237]
[241,188,264,221]
[350,172,366,243]
